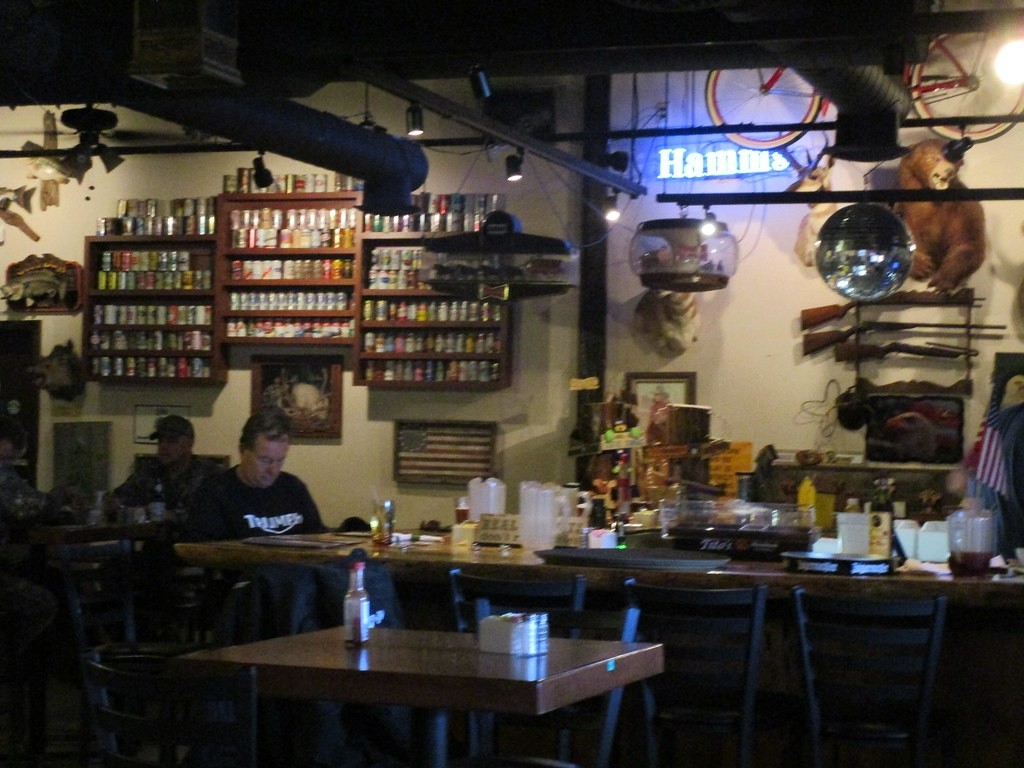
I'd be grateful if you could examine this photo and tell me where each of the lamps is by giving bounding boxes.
[603,188,625,224]
[404,102,427,137]
[941,125,973,163]
[504,147,529,184]
[96,137,129,174]
[249,151,277,188]
[60,142,94,186]
[466,56,493,101]
[698,203,721,242]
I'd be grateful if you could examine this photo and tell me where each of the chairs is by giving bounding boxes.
[444,562,593,759]
[17,531,139,754]
[620,581,778,768]
[439,591,646,768]
[125,449,230,587]
[782,583,962,768]
[76,646,262,768]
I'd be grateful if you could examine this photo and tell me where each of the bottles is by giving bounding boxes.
[345,562,369,647]
[229,317,355,339]
[149,477,166,516]
[523,614,548,655]
[224,168,364,192]
[365,193,501,232]
[231,210,356,247]
[361,330,499,382]
[363,247,502,321]
[98,197,215,235]
[798,478,815,508]
[233,259,353,280]
[846,497,861,514]
[94,357,203,377]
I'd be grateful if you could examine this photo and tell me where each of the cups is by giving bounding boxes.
[558,484,591,537]
[453,497,471,524]
[519,481,570,553]
[838,512,870,555]
[947,510,992,575]
[371,500,394,544]
[467,478,507,523]
[868,513,891,557]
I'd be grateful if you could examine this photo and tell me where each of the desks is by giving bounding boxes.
[169,620,668,768]
[26,502,175,607]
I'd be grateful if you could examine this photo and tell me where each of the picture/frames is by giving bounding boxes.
[251,352,345,439]
[624,369,700,405]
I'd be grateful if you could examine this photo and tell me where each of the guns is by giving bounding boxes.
[800,288,1009,363]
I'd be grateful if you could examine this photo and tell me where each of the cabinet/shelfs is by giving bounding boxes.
[217,186,362,345]
[75,228,225,387]
[351,229,515,391]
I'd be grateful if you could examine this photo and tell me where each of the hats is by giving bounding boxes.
[150,415,194,440]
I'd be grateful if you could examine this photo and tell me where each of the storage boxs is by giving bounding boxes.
[674,525,820,555]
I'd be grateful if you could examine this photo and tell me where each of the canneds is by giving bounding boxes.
[226,317,355,338]
[89,304,213,378]
[97,250,213,290]
[223,166,327,193]
[96,195,216,236]
[226,207,355,311]
[364,193,501,233]
[362,249,501,383]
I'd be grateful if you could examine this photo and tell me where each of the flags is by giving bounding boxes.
[962,384,1013,504]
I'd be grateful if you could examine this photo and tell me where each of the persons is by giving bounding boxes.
[0,419,74,664]
[103,415,224,552]
[193,406,326,543]
